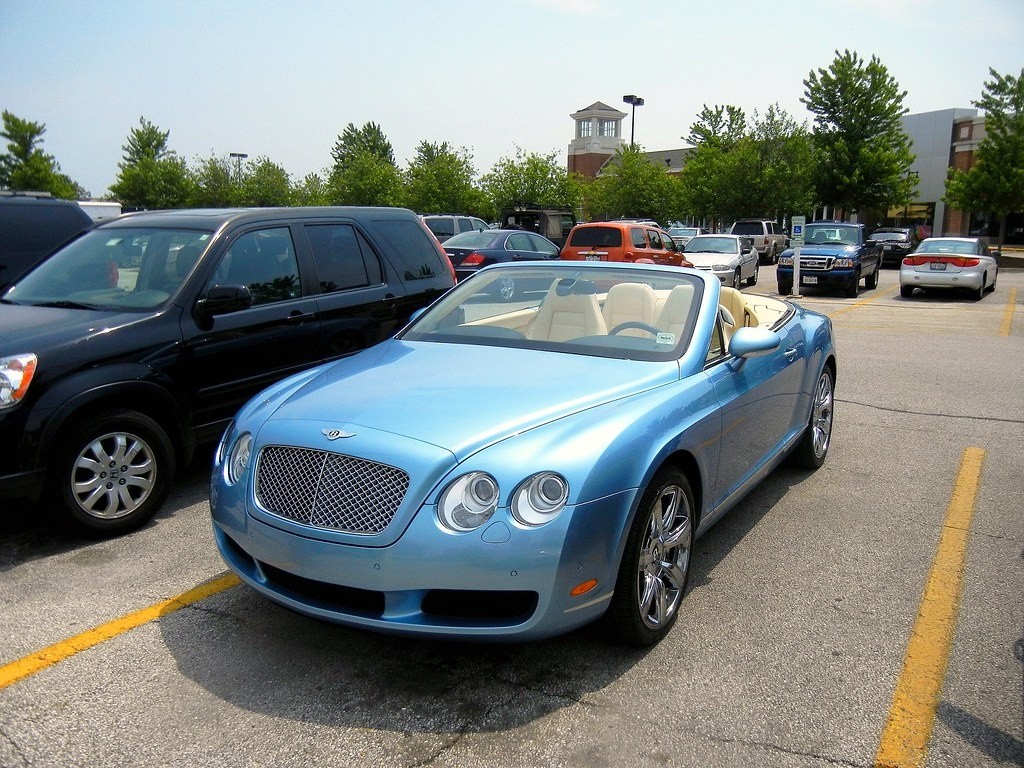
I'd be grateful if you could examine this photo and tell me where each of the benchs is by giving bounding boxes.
[602,283,745,354]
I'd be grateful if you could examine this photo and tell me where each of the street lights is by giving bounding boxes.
[230,152,248,188]
[623,95,644,147]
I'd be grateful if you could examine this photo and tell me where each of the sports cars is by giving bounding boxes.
[210,260,838,642]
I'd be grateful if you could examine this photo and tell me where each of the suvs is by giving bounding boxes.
[417,213,492,244]
[730,219,788,265]
[489,206,710,268]
[0,191,465,535]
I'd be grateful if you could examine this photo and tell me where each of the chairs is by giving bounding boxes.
[843,230,856,244]
[814,232,829,243]
[226,236,294,306]
[531,278,608,343]
[649,284,746,356]
[171,246,211,297]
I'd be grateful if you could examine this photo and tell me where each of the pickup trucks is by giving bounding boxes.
[776,220,883,298]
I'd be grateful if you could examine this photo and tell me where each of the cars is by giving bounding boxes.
[441,229,562,303]
[680,234,759,290]
[900,237,1001,300]
[869,227,922,269]
